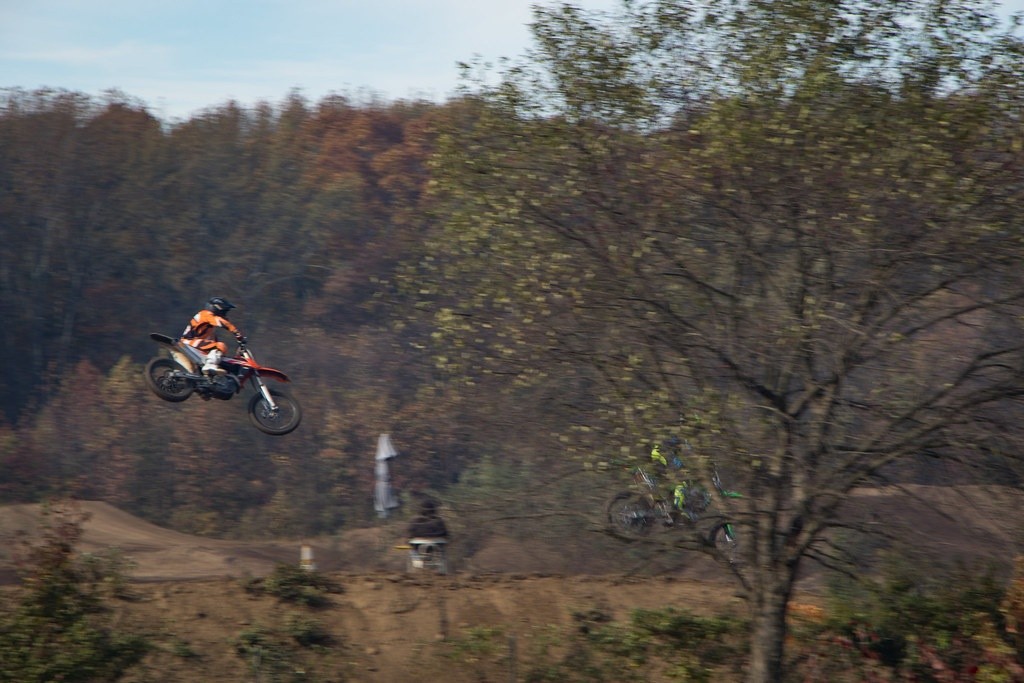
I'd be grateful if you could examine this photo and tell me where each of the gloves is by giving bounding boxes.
[238,337,247,347]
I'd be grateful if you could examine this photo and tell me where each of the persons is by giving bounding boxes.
[406,501,449,557]
[180,297,243,377]
[633,433,695,524]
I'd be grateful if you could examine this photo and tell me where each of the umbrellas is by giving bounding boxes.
[373,430,399,520]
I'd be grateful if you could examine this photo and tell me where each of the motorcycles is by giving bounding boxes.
[143,332,302,435]
[602,458,759,568]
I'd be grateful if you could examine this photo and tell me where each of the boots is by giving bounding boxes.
[201,349,227,376]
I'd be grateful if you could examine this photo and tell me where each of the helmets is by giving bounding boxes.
[205,297,236,316]
